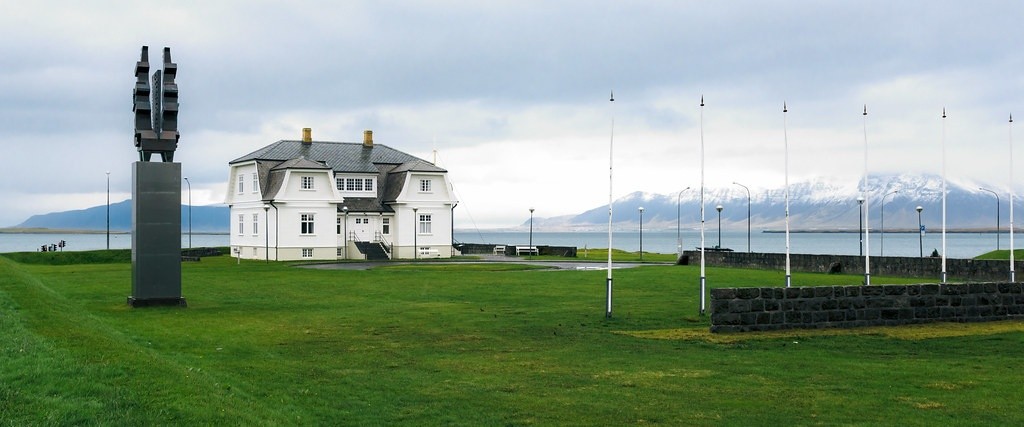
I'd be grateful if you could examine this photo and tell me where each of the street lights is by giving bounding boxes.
[715,205,723,248]
[412,207,418,260]
[263,204,271,264]
[638,207,643,261]
[915,206,924,257]
[881,190,899,257]
[342,206,348,261]
[678,186,691,239]
[184,177,191,249]
[528,208,535,258]
[106,171,110,250]
[856,196,866,257]
[733,182,750,253]
[979,187,1000,250]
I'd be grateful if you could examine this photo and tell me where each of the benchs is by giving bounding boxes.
[516,246,539,256]
[418,249,440,259]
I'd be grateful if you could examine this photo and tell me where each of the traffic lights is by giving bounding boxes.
[41,245,44,251]
[62,241,66,248]
[54,244,57,251]
